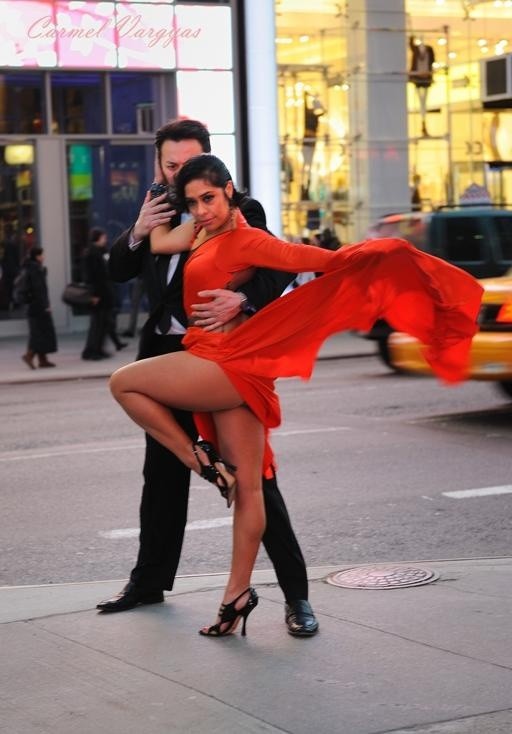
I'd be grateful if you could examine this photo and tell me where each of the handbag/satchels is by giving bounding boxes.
[62,283,94,305]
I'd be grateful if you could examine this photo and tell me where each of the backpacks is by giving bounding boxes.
[12,268,38,305]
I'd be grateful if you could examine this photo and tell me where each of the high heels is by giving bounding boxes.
[193,440,238,507]
[199,586,258,636]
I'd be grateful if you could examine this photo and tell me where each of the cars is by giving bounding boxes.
[385,263,512,402]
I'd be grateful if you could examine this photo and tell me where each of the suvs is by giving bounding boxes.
[357,201,512,373]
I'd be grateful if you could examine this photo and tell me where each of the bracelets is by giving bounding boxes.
[149,181,170,204]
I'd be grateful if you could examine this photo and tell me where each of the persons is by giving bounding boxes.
[409,32,439,137]
[79,213,345,361]
[15,246,62,369]
[111,138,401,640]
[298,87,326,200]
[96,120,325,637]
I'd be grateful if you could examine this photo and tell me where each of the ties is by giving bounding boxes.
[156,252,186,334]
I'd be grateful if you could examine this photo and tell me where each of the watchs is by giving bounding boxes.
[238,292,252,311]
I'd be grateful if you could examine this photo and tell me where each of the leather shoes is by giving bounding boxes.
[283,599,320,635]
[95,581,165,611]
[82,351,102,359]
[117,343,127,350]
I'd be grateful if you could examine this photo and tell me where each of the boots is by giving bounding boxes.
[39,356,55,367]
[23,351,35,369]
